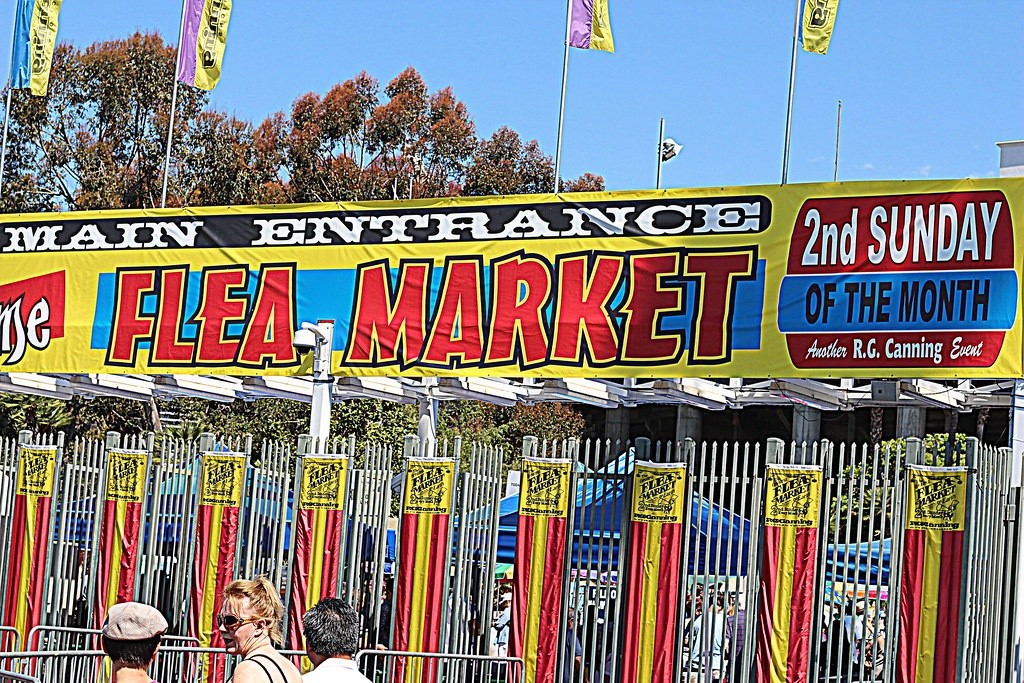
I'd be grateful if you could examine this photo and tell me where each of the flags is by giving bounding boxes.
[179,0,233,90]
[797,0,839,53]
[569,0,614,53]
[10,0,61,97]
[662,138,684,163]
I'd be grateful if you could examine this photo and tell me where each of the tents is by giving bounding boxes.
[53,443,892,582]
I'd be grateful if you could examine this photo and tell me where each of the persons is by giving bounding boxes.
[29,563,886,683]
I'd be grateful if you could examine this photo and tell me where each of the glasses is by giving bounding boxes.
[216,613,260,629]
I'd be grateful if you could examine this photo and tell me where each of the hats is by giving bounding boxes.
[101,602,168,640]
[856,601,872,612]
[500,592,512,604]
